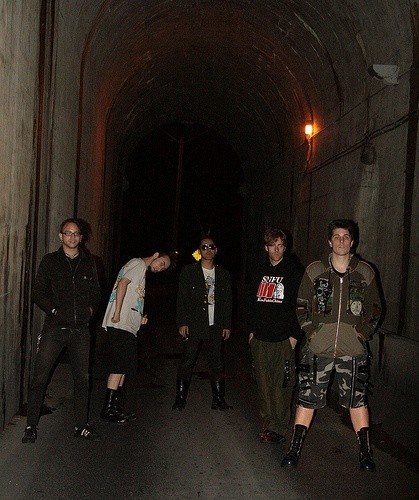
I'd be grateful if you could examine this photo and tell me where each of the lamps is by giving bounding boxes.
[367,63,400,86]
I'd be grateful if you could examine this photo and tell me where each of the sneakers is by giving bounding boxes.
[22,425,37,443]
[74,423,101,441]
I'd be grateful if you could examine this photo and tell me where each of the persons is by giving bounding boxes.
[172,235,233,411]
[242,225,307,444]
[20,218,103,444]
[279,218,386,474]
[96,249,172,423]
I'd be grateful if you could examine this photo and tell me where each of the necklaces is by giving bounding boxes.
[65,252,79,257]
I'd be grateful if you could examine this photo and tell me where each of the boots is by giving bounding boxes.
[99,388,128,424]
[281,424,309,468]
[356,427,376,471]
[210,378,230,411]
[172,378,192,410]
[113,386,130,419]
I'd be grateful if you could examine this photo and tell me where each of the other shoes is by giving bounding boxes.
[256,429,286,443]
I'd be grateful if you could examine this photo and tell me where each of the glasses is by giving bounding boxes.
[61,231,82,236]
[200,244,216,250]
